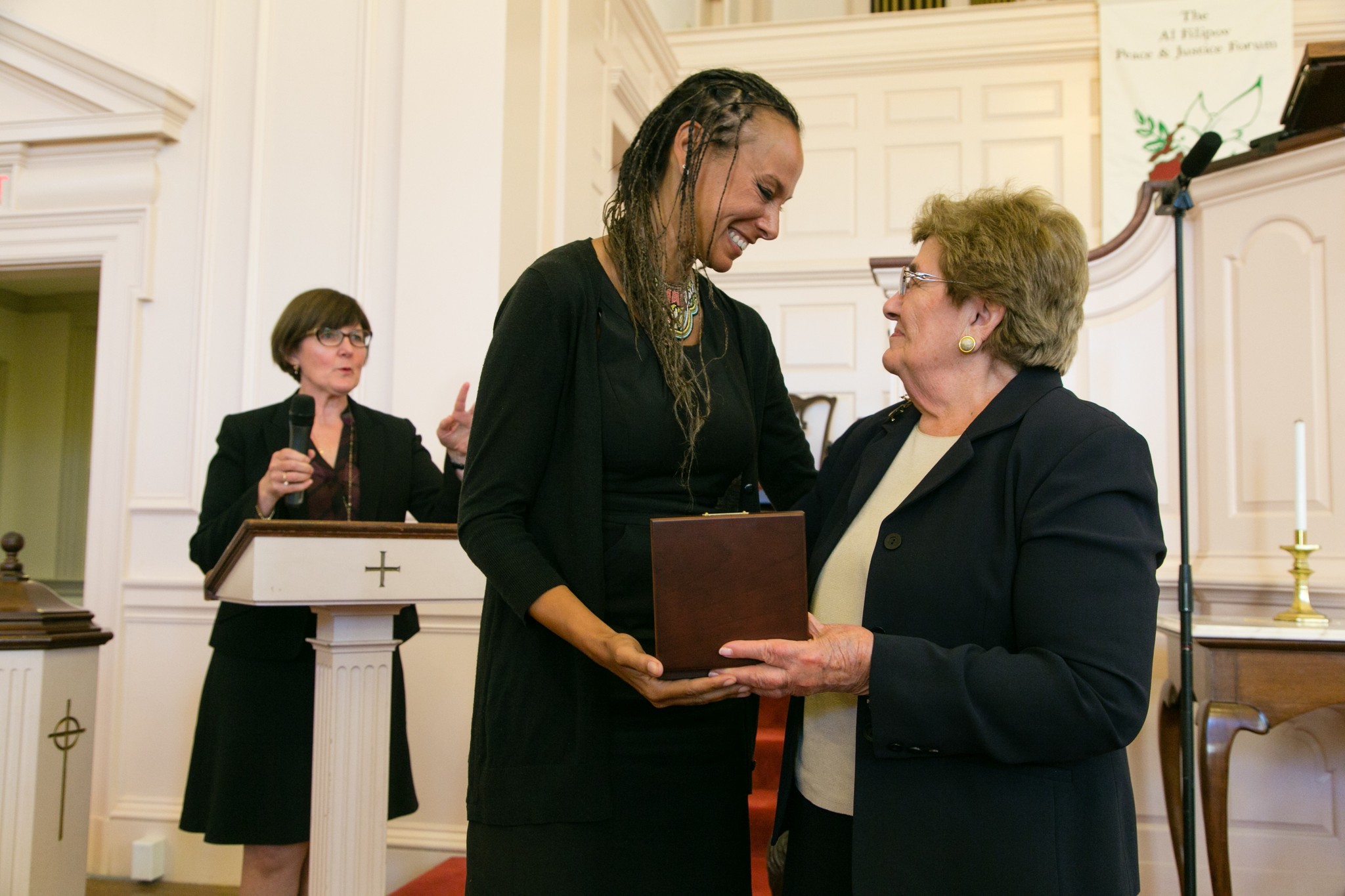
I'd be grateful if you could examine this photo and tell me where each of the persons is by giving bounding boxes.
[708,179,1168,896]
[457,69,817,895]
[178,287,473,896]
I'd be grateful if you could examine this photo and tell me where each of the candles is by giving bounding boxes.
[1292,420,1307,529]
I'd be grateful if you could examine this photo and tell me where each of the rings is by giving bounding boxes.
[283,471,286,482]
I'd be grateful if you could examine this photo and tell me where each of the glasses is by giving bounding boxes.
[299,327,373,348]
[900,264,974,298]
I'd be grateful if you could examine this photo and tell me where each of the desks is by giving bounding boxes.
[1154,615,1344,896]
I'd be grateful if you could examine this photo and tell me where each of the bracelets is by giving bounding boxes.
[451,462,465,470]
[256,505,274,520]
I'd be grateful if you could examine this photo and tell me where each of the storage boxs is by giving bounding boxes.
[649,508,808,678]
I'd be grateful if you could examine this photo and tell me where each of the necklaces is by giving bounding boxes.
[655,268,698,339]
[310,397,354,521]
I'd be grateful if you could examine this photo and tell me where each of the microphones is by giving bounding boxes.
[286,393,317,517]
[1149,131,1224,217]
